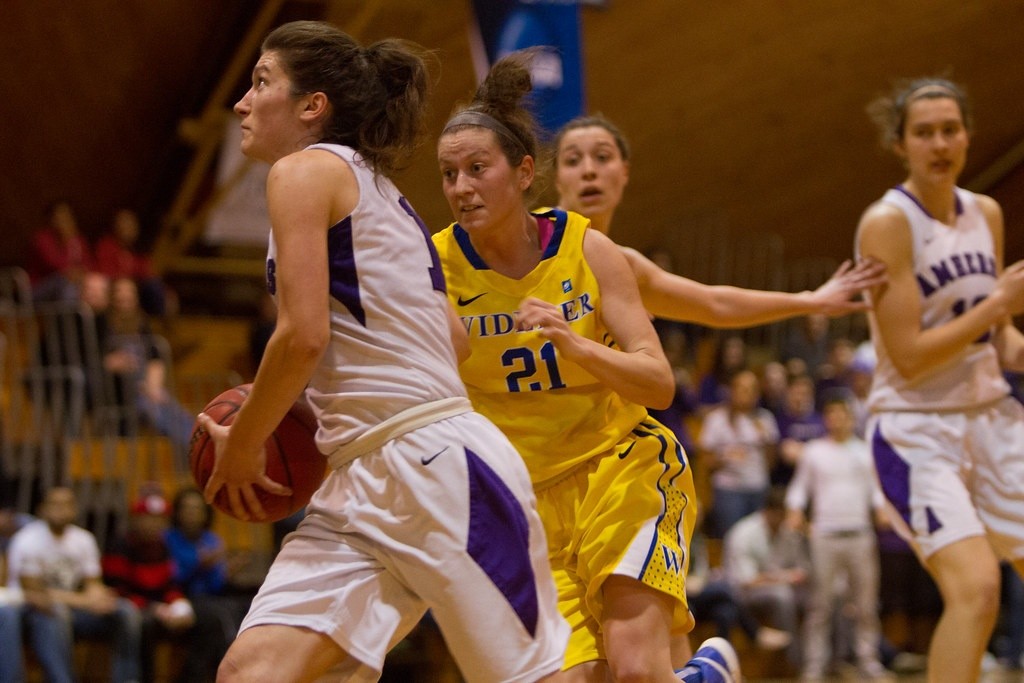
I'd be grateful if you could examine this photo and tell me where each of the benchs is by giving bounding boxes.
[0,312,799,683]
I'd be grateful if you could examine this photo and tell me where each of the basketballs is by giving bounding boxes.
[186,380,327,526]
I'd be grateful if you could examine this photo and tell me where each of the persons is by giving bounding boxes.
[646,311,878,683]
[554,116,888,672]
[855,78,1024,683]
[8,487,226,683]
[198,22,572,683]
[16,202,197,435]
[431,45,741,683]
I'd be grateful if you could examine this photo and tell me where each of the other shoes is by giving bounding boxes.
[804,660,829,682]
[859,657,897,681]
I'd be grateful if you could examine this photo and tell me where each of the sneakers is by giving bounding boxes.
[672,636,743,683]
